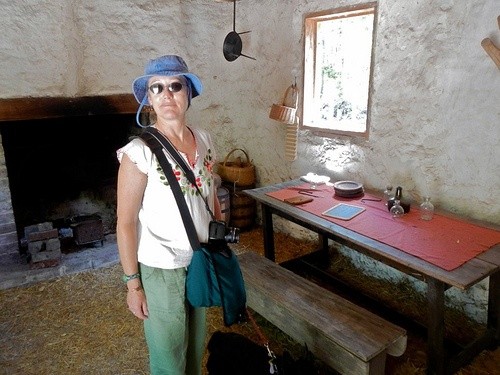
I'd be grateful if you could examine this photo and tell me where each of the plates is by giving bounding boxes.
[333,181,364,198]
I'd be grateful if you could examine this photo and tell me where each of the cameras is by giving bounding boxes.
[208,220,240,244]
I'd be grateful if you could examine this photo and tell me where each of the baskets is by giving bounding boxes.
[269,85,299,125]
[217,147,256,188]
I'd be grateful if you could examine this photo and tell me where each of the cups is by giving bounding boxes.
[310,178,317,189]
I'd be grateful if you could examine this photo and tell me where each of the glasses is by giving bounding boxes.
[147,82,186,94]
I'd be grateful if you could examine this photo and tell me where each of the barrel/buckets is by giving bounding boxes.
[220,181,256,230]
[217,186,230,227]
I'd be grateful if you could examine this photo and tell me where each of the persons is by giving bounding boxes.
[116,54,222,375]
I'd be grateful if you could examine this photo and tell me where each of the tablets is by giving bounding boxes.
[321,203,365,220]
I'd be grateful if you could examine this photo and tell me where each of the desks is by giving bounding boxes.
[241,178,500,375]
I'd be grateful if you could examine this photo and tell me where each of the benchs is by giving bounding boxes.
[237,252,408,375]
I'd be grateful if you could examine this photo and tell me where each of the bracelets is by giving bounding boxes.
[128,285,143,291]
[122,273,140,280]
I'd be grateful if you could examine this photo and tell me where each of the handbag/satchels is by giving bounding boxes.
[185,242,247,327]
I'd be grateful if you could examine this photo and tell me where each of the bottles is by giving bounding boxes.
[420,195,434,220]
[390,200,404,218]
[383,185,395,205]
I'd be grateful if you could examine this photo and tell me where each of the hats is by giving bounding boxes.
[132,55,203,107]
